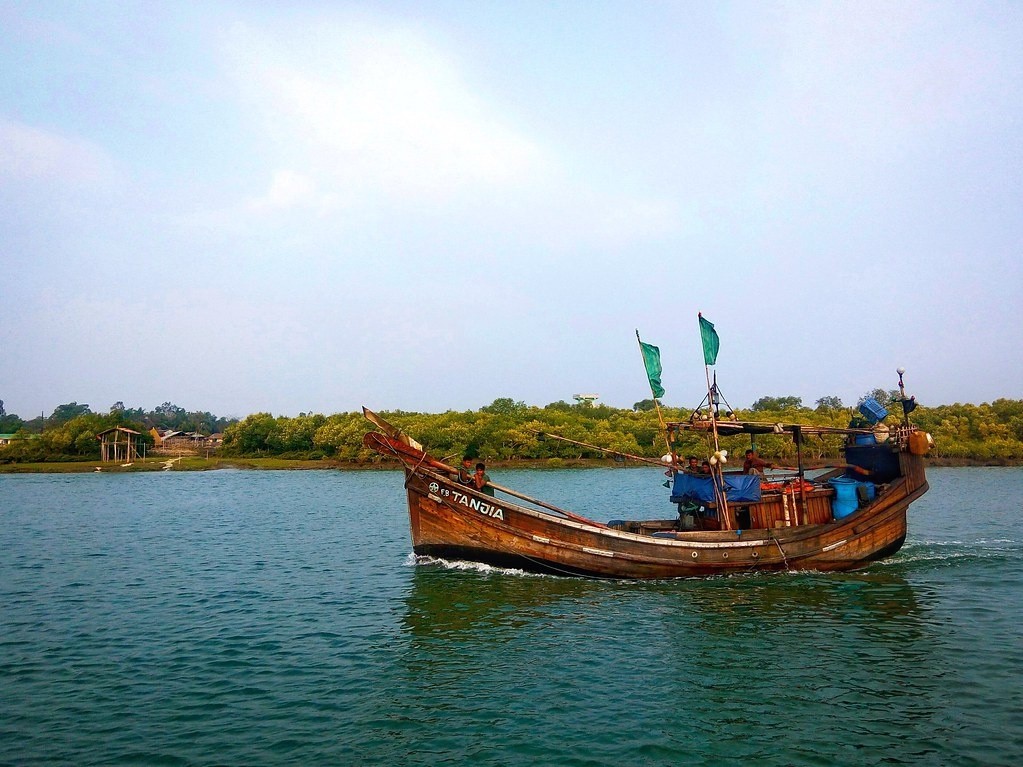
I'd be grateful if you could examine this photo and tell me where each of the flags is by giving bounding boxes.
[699,316,719,365]
[640,342,665,398]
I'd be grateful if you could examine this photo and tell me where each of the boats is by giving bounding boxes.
[362,367,934,580]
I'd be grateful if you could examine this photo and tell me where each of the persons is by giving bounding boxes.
[474,463,494,497]
[457,455,474,489]
[685,458,701,474]
[699,461,716,475]
[743,450,780,475]
